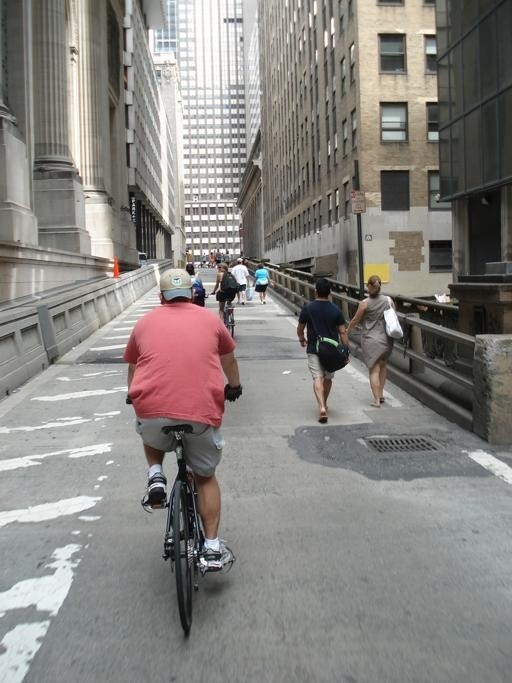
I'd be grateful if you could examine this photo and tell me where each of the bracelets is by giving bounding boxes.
[299,339,305,342]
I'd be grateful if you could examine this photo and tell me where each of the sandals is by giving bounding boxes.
[317,405,330,424]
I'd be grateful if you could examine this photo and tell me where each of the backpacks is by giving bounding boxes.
[220,271,240,293]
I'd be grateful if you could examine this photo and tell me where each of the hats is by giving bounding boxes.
[237,257,243,262]
[158,267,195,301]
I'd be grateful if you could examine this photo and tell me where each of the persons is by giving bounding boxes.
[202,249,231,265]
[123,267,241,571]
[252,263,273,304]
[346,275,396,406]
[212,262,236,323]
[296,278,350,423]
[186,264,206,307]
[231,257,249,304]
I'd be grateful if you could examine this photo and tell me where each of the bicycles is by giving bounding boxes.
[126,386,245,637]
[211,291,236,338]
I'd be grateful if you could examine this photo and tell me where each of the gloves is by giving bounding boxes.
[224,382,242,402]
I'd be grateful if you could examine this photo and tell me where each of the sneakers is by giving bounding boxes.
[236,299,245,305]
[139,470,170,508]
[200,541,235,572]
[259,299,267,305]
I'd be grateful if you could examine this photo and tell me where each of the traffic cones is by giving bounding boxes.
[112,255,121,278]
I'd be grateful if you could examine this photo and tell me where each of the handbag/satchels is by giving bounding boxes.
[382,309,405,339]
[314,335,351,373]
